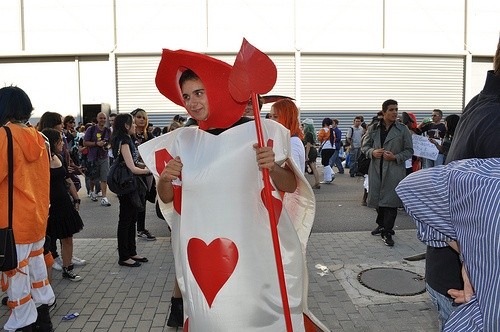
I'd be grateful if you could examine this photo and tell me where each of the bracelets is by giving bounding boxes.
[459,254,462,264]
[94,141,98,146]
[73,199,81,205]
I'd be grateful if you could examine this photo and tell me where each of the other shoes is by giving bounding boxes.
[72,256,86,265]
[350,173,363,177]
[370,224,386,235]
[320,176,335,184]
[381,231,394,245]
[52,262,62,271]
[118,257,148,268]
[100,197,111,206]
[90,191,98,201]
[312,186,320,189]
[137,229,156,241]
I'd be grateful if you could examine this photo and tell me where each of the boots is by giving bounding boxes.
[166,296,184,328]
[362,188,368,206]
[14,305,53,332]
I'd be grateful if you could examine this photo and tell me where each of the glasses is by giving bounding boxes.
[431,114,440,116]
[69,121,76,124]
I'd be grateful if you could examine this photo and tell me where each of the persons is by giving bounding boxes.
[425,34,500,332]
[360,99,414,246]
[394,158,500,332]
[38,111,83,269]
[110,113,149,267]
[26,112,188,205]
[130,109,157,240]
[243,95,460,210]
[40,129,81,280]
[0,86,56,332]
[166,118,198,327]
[138,49,332,332]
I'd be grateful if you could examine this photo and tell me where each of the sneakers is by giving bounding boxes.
[62,264,81,281]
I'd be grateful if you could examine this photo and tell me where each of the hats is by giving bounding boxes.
[304,118,313,124]
[402,111,414,126]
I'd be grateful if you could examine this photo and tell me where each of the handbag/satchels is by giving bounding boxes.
[62,144,82,196]
[318,146,322,153]
[107,141,138,195]
[356,122,378,175]
[0,227,17,271]
